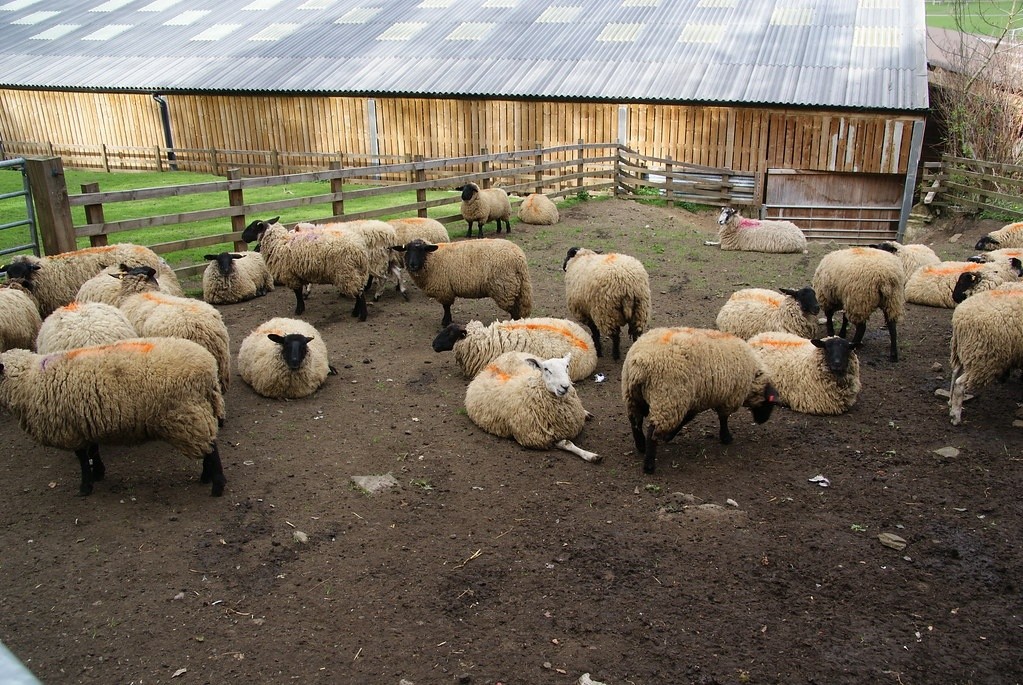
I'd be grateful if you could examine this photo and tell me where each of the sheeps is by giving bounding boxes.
[744,331,863,415]
[517,193,559,225]
[455,183,512,238]
[946,282,1023,425]
[719,288,821,343]
[237,316,339,397]
[242,215,452,322]
[388,237,534,326]
[432,318,598,384]
[469,354,604,463]
[0,337,227,500]
[564,247,653,362]
[703,207,807,254]
[815,250,904,364]
[868,221,1023,308]
[109,261,230,395]
[629,331,783,472]
[0,244,187,354]
[202,251,273,305]
